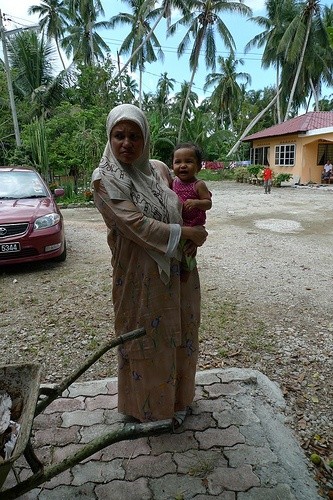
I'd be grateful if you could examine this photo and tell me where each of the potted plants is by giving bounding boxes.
[235,164,293,187]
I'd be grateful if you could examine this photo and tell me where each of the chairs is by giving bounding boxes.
[320,170,333,184]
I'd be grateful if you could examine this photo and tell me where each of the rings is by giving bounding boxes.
[194,247,197,251]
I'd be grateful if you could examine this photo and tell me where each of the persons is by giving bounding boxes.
[167,143,212,282]
[201,159,236,170]
[90,103,209,424]
[321,160,333,184]
[260,162,274,194]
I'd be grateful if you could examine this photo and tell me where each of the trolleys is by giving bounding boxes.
[0,329,173,500]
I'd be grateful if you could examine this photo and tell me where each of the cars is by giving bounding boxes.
[0,164,67,268]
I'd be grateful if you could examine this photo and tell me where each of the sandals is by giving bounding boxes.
[173,410,187,430]
